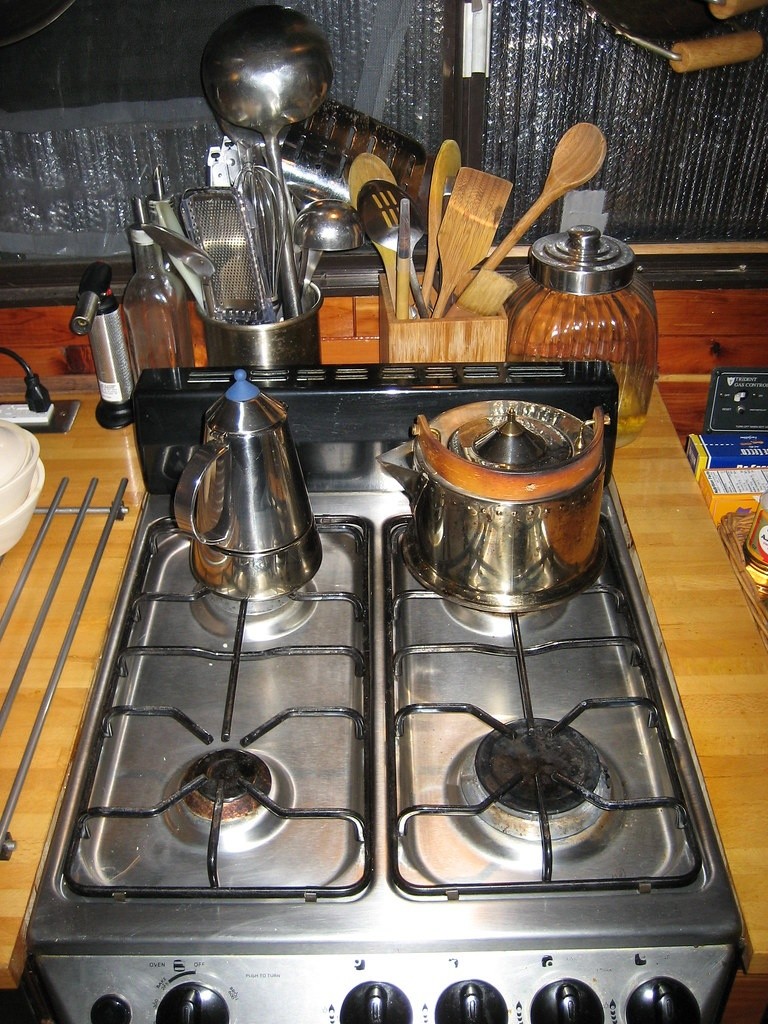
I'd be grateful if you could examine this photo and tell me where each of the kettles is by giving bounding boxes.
[175,368,322,601]
[376,400,610,613]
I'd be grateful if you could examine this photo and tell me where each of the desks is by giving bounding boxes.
[0,354,767,1024]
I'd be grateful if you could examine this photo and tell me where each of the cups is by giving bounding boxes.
[196,280,323,369]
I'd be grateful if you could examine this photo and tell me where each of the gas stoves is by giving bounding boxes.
[25,359,742,1023]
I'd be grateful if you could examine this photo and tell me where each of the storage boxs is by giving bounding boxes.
[376,272,512,365]
[686,430,768,523]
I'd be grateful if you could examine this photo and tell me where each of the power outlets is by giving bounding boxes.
[0,404,55,427]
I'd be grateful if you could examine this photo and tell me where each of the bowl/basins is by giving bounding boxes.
[0,420,45,556]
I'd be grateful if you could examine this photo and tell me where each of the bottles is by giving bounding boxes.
[147,193,195,299]
[123,222,194,377]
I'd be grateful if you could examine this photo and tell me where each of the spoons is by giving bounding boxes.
[481,123,606,271]
[141,224,219,319]
